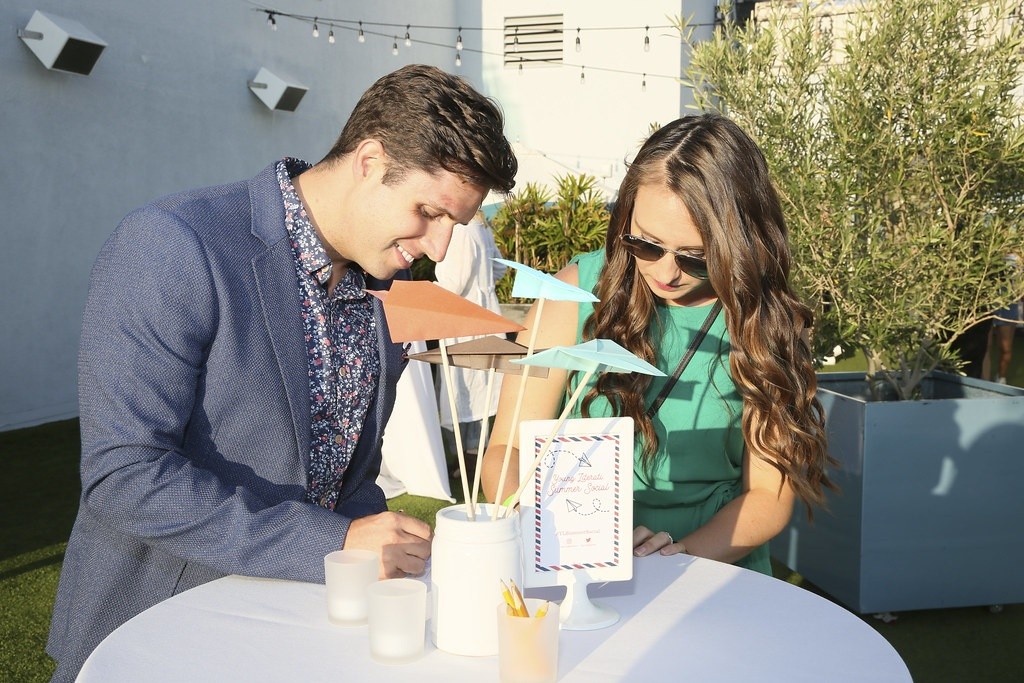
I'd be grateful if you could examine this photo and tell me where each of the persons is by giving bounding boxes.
[481,114,819,585]
[52,58,518,683]
[428,205,508,480]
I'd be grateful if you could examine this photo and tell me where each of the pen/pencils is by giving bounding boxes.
[498,576,551,618]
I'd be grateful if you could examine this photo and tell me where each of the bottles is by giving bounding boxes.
[431,503,523,656]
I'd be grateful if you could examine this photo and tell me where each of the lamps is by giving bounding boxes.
[17,10,108,77]
[247,67,308,113]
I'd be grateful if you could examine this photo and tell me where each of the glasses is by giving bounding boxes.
[619,215,712,281]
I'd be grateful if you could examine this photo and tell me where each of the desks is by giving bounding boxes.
[73,534,914,682]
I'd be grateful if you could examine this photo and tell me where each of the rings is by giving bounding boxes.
[659,530,674,543]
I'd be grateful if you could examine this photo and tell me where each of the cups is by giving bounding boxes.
[368,578,427,662]
[324,550,380,628]
[497,598,560,683]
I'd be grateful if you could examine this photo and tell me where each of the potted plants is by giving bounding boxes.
[647,0,1023,624]
